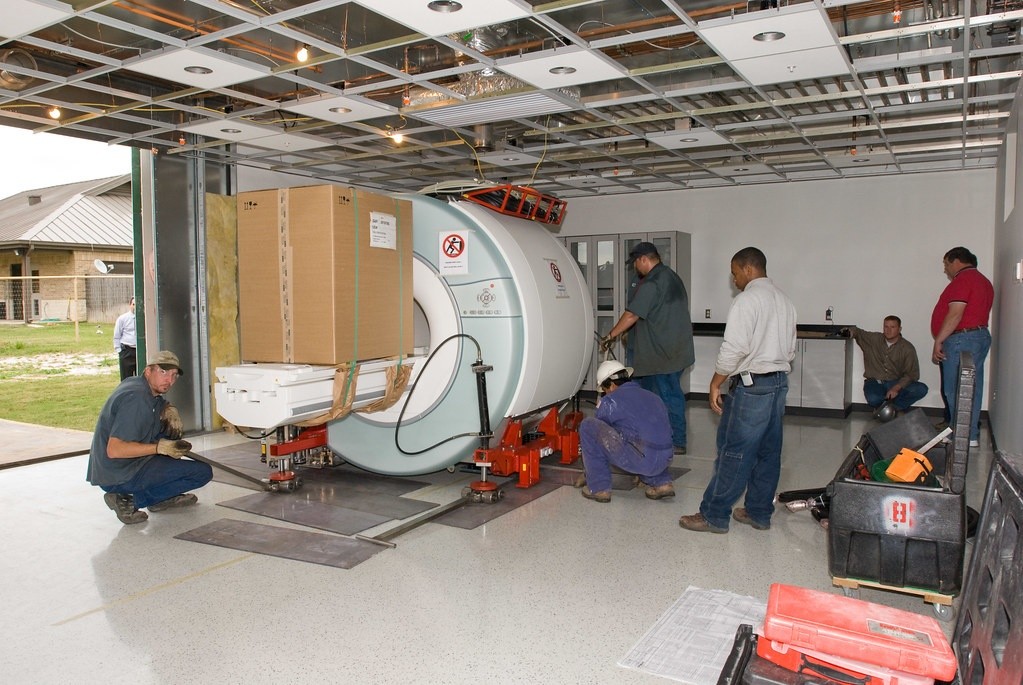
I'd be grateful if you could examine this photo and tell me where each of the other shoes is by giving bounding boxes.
[936,422,948,429]
[968,439,979,447]
[673,447,687,455]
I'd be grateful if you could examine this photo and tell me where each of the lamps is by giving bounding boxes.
[13,249,25,256]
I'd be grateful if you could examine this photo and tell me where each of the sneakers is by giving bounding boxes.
[644,483,675,499]
[680,513,729,534]
[147,493,198,512]
[582,486,611,502]
[733,508,771,530]
[104,492,148,524]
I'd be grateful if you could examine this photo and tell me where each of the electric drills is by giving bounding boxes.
[786,494,829,530]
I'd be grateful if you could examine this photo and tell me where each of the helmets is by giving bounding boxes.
[597,360,634,392]
[873,399,898,422]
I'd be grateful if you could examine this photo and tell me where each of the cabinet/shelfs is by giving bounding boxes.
[556,231,694,402]
[785,339,854,418]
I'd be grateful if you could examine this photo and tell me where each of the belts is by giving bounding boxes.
[752,372,777,378]
[951,326,979,334]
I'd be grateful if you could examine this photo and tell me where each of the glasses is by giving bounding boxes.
[156,365,179,379]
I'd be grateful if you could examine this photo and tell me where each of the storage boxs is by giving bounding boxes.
[716,448,1023,685]
[756,624,935,685]
[235,183,414,368]
[830,353,977,597]
[764,582,957,681]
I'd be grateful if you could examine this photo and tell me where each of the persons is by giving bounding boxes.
[929,247,996,447]
[600,240,695,454]
[581,360,675,503]
[114,296,137,381]
[675,246,799,533]
[840,315,928,420]
[86,350,215,525]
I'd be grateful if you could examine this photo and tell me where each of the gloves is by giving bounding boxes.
[160,407,181,440]
[156,438,192,459]
[575,473,586,488]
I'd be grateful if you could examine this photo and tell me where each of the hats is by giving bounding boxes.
[149,351,184,376]
[625,242,656,264]
